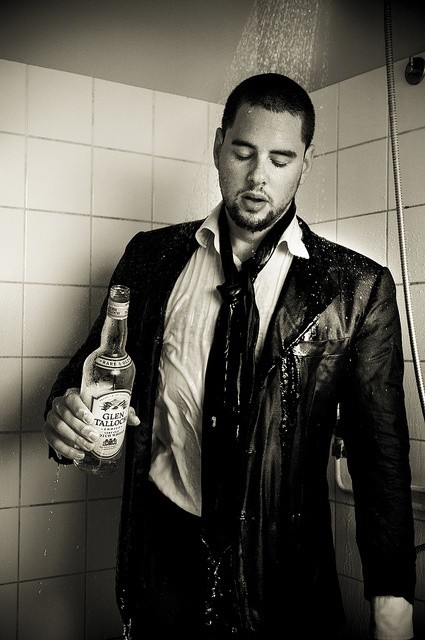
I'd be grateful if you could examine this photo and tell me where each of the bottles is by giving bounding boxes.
[72,284,136,477]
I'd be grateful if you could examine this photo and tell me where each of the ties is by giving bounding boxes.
[200,198,295,560]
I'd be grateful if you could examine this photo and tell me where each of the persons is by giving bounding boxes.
[40,72,416,640]
